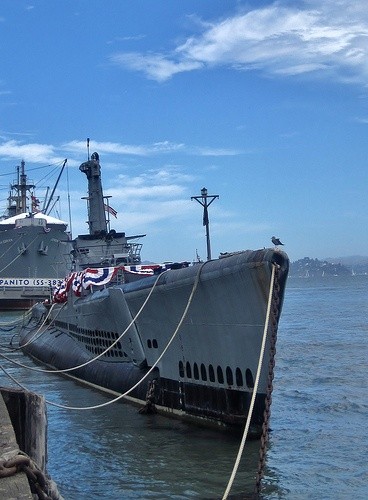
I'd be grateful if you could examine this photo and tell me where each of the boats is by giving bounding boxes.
[14,184,294,444]
[64,136,148,271]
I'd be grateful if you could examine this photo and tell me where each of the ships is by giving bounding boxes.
[0,158,75,314]
